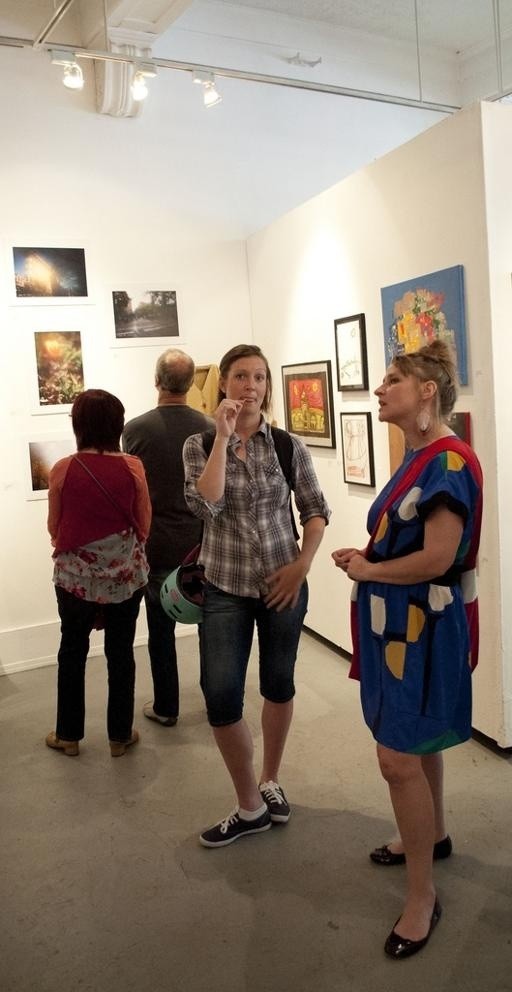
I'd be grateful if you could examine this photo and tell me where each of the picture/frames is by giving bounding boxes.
[332,313,370,393]
[26,316,95,415]
[104,278,187,351]
[388,410,473,484]
[379,263,472,389]
[11,244,88,300]
[336,409,376,489]
[22,427,78,504]
[280,358,337,451]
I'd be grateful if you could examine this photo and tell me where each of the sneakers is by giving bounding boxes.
[199,809,270,847]
[142,700,179,728]
[258,781,293,827]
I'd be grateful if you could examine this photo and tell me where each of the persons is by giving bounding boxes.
[124,349,218,726]
[46,388,152,757]
[333,342,484,960]
[181,344,330,847]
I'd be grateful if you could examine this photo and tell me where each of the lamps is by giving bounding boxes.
[125,64,147,101]
[59,59,82,92]
[203,75,224,103]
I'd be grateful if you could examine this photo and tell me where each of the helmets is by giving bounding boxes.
[160,565,206,627]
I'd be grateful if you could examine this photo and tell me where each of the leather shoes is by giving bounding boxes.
[370,835,454,868]
[382,895,443,959]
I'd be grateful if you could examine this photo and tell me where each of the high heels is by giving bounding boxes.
[45,729,80,759]
[109,730,140,758]
